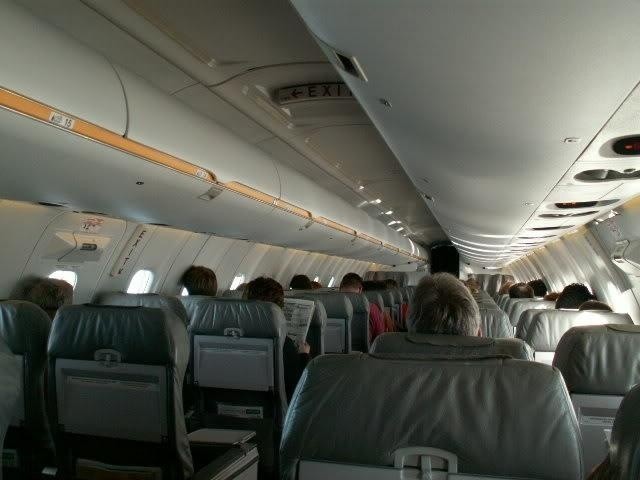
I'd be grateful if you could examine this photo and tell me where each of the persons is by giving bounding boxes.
[499,280,612,311]
[180,265,322,378]
[340,271,406,341]
[26,278,75,322]
[406,272,482,337]
